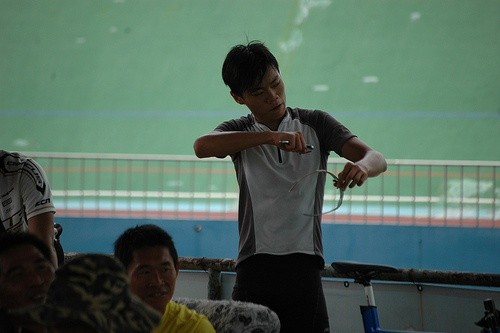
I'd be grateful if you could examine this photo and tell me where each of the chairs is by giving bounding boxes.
[170,297,281,333]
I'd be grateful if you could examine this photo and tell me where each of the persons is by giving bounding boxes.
[0,149,215,333]
[194,38,386,333]
